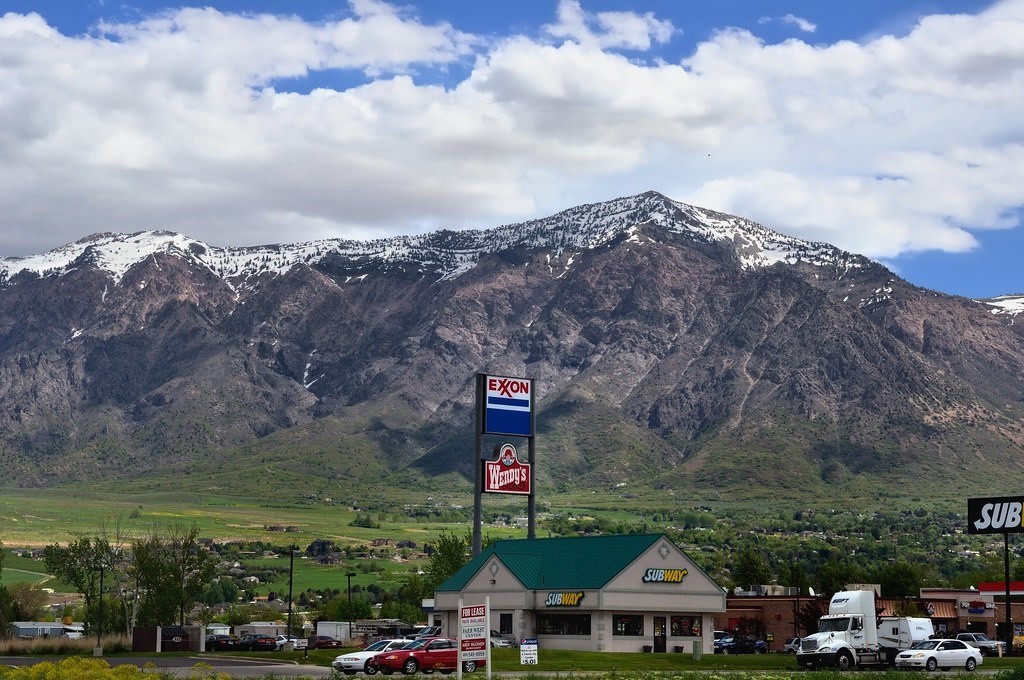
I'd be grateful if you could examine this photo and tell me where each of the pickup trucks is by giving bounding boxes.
[932,629,1005,653]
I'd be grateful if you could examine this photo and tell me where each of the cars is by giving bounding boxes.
[239,633,277,650]
[204,633,238,652]
[405,626,443,637]
[891,637,984,672]
[488,628,517,649]
[370,636,489,673]
[715,636,769,655]
[329,639,416,675]
[313,633,342,648]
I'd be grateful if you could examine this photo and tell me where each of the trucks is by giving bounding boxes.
[277,633,309,651]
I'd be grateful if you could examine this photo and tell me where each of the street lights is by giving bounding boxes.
[345,568,356,642]
[794,559,804,635]
[93,563,104,656]
[287,542,298,653]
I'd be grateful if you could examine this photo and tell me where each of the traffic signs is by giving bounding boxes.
[458,599,488,660]
